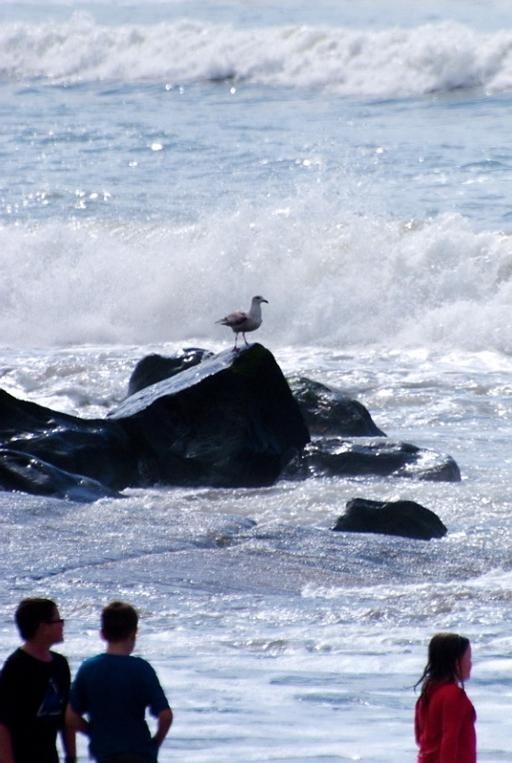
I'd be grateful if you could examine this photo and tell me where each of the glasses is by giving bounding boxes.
[44,619,64,624]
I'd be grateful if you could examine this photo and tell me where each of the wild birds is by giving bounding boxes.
[215,295,269,348]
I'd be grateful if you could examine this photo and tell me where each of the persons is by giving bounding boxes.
[64,601,173,763]
[0,598,77,762]
[414,631,476,762]
